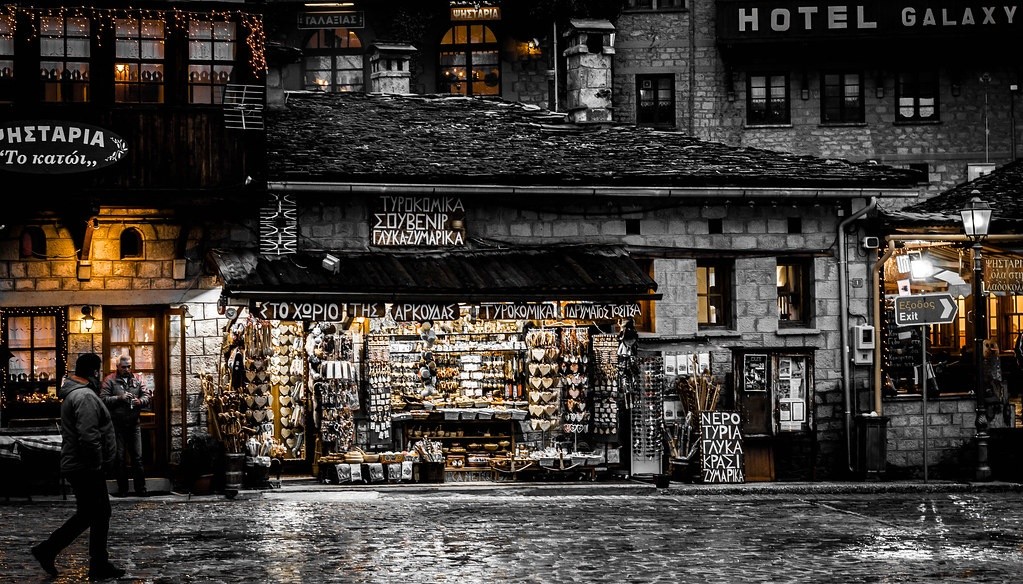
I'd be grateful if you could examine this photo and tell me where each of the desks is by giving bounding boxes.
[0,433,67,500]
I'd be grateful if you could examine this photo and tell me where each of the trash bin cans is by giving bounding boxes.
[854,414,890,482]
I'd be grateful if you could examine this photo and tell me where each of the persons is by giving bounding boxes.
[30,352,127,578]
[101,354,151,497]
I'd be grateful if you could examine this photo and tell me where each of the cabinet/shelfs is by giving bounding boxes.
[271,320,664,481]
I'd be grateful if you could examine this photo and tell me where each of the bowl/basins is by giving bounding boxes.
[468,462,489,467]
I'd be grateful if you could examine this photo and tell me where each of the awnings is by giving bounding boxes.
[227,255,663,301]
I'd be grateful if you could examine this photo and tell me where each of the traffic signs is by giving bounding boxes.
[895,294,958,327]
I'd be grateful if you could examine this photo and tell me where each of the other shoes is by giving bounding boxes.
[88,564,127,579]
[136,490,151,497]
[115,491,128,496]
[31,545,59,577]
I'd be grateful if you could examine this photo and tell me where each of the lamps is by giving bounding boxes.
[81,306,95,332]
[181,305,193,328]
[322,253,340,275]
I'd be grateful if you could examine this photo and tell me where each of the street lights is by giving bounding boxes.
[958,188,994,478]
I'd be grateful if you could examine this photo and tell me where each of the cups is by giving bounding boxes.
[128,395,136,403]
